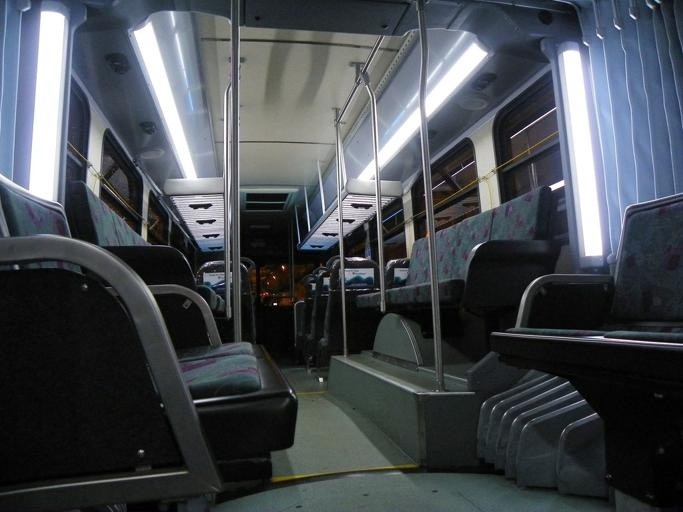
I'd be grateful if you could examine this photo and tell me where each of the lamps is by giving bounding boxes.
[132,16,198,178]
[356,41,489,181]
[28,1,70,203]
[558,40,605,269]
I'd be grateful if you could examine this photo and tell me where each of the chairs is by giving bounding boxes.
[308,254,346,340]
[356,232,435,310]
[319,259,378,347]
[304,266,326,325]
[385,258,409,289]
[196,260,256,343]
[213,295,225,313]
[369,208,495,310]
[305,271,331,340]
[240,256,255,271]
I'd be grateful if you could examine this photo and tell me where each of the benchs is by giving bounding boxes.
[414,186,552,313]
[64,180,218,312]
[0,173,299,512]
[489,193,683,383]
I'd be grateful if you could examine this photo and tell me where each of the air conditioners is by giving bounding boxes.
[239,189,298,214]
